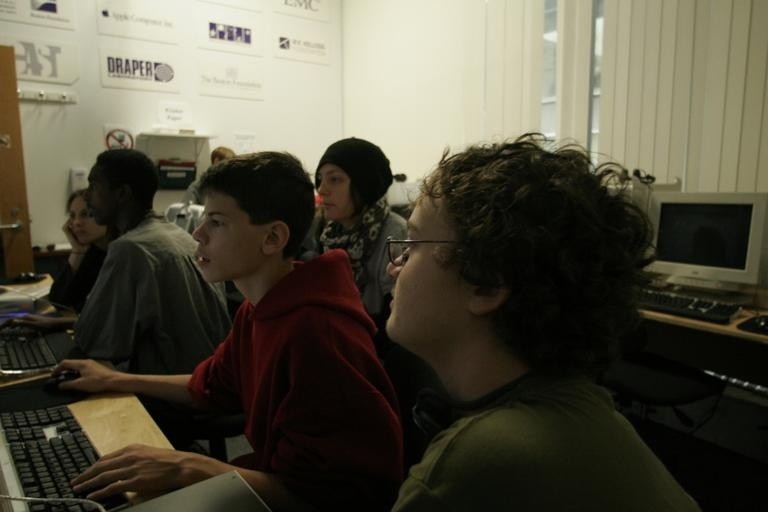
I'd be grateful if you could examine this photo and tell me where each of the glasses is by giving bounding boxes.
[384,233,454,266]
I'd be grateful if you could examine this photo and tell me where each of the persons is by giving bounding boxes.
[1,146,235,456]
[384,132,704,510]
[49,150,406,512]
[181,144,238,205]
[293,136,410,315]
[47,186,108,316]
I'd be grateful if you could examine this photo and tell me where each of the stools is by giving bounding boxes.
[602,352,722,442]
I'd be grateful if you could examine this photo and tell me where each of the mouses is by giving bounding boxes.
[746,317,767,329]
[45,367,81,396]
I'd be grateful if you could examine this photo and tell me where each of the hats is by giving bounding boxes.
[315,137,393,201]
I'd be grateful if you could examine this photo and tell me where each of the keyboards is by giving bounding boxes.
[0,405,129,512]
[637,288,743,325]
[0,330,59,380]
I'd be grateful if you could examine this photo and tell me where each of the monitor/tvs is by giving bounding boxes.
[642,192,768,291]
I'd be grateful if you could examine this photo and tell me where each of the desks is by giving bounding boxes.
[629,304,768,345]
[1,273,187,512]
